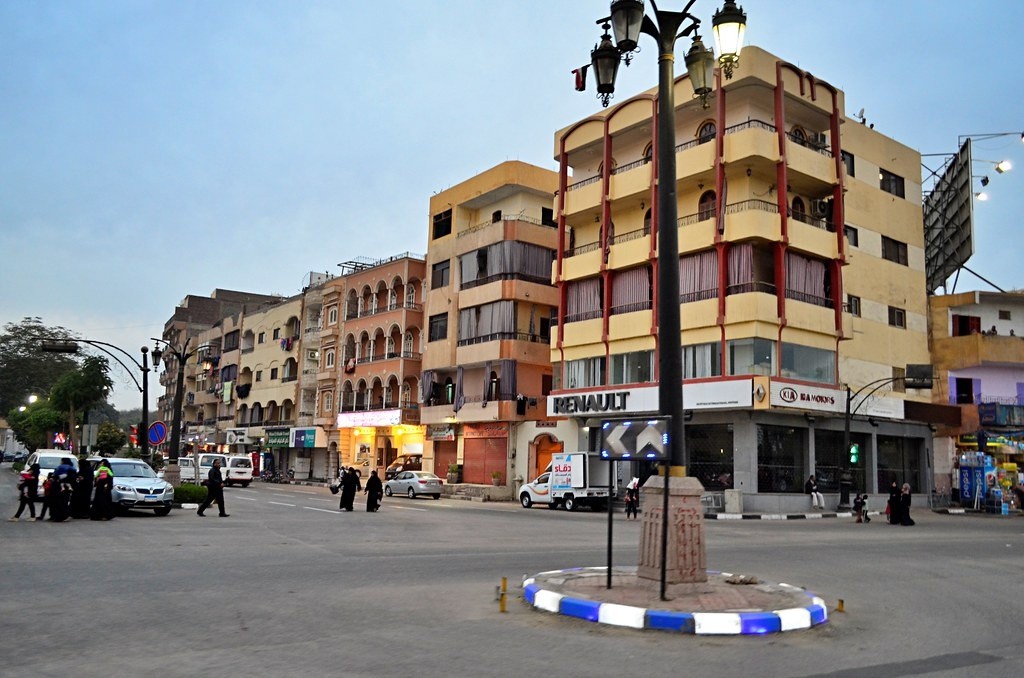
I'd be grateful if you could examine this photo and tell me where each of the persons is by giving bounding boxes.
[339,466,346,490]
[972,325,997,336]
[885,481,915,526]
[860,119,866,125]
[805,475,824,510]
[197,459,230,517]
[853,493,871,523]
[1010,329,1015,336]
[870,124,874,129]
[364,471,383,512]
[1009,485,1024,515]
[406,459,412,464]
[625,477,639,520]
[8,458,114,522]
[337,467,361,510]
[261,469,273,483]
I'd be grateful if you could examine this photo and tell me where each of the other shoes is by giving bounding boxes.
[219,514,229,517]
[6,516,19,522]
[197,510,205,516]
[25,517,37,522]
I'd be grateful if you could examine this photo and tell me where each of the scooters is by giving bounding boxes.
[260,463,285,484]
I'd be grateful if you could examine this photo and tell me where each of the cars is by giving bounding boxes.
[383,469,445,500]
[18,449,81,499]
[85,454,176,516]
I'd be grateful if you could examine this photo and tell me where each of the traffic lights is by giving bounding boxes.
[128,423,142,445]
[848,443,859,466]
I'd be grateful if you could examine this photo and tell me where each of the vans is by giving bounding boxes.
[197,453,255,487]
[384,453,423,480]
[157,457,203,485]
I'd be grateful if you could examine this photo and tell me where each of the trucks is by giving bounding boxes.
[518,451,619,511]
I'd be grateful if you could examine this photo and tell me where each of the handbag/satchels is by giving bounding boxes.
[329,484,339,495]
[885,502,891,514]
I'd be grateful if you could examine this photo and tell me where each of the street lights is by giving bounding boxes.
[570,0,750,584]
[150,336,220,488]
